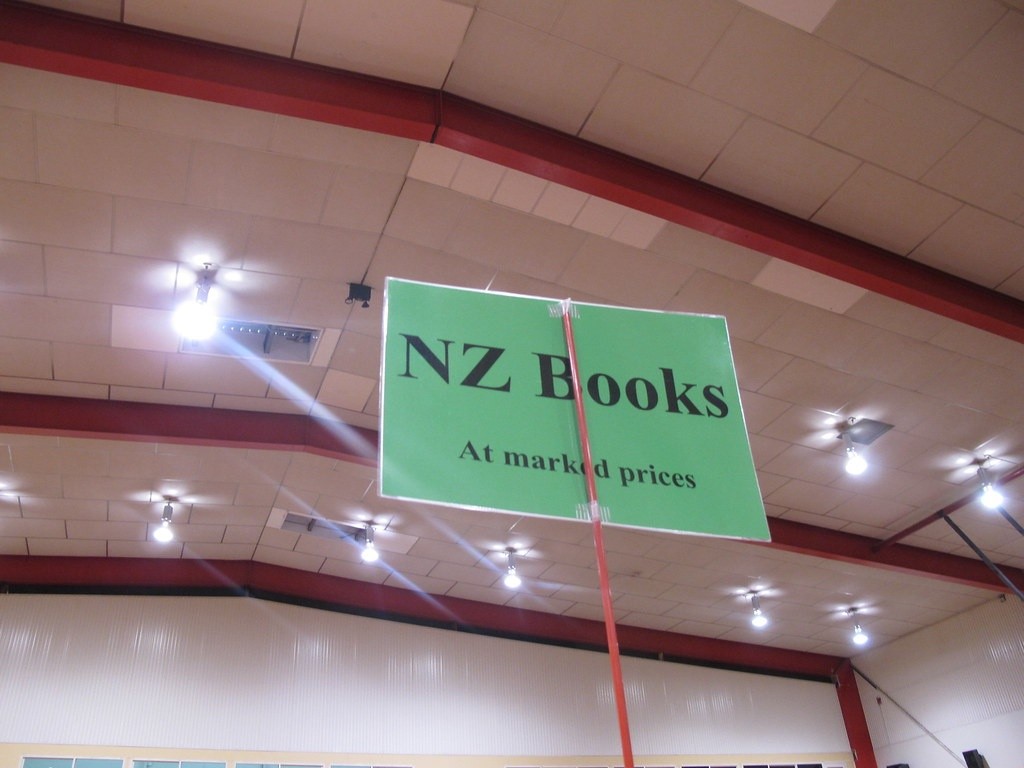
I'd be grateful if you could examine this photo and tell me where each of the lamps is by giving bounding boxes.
[748,589,769,629]
[849,605,870,648]
[359,522,379,564]
[343,282,372,309]
[835,413,868,480]
[165,267,224,345]
[969,458,1005,514]
[153,494,179,541]
[503,550,523,589]
